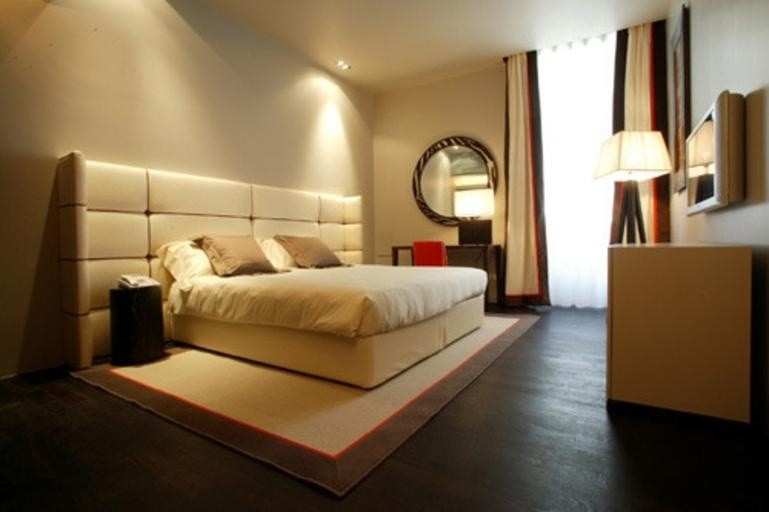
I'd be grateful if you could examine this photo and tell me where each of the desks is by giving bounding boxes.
[390,245,505,313]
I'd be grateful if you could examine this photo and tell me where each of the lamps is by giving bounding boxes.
[584,129,673,243]
[452,184,494,246]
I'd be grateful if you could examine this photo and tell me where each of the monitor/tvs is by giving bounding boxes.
[685,89,746,217]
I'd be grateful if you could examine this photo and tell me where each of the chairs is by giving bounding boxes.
[412,241,447,266]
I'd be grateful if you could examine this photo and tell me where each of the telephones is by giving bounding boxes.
[117,274,160,289]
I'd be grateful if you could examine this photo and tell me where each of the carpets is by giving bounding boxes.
[70,313,558,497]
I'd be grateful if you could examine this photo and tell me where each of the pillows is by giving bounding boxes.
[194,236,343,276]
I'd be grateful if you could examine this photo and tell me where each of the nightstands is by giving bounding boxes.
[109,290,164,362]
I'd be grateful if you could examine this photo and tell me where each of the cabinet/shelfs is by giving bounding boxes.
[607,244,750,427]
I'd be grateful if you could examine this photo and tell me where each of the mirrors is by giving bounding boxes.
[411,136,499,225]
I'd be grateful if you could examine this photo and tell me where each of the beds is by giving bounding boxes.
[165,236,487,389]
